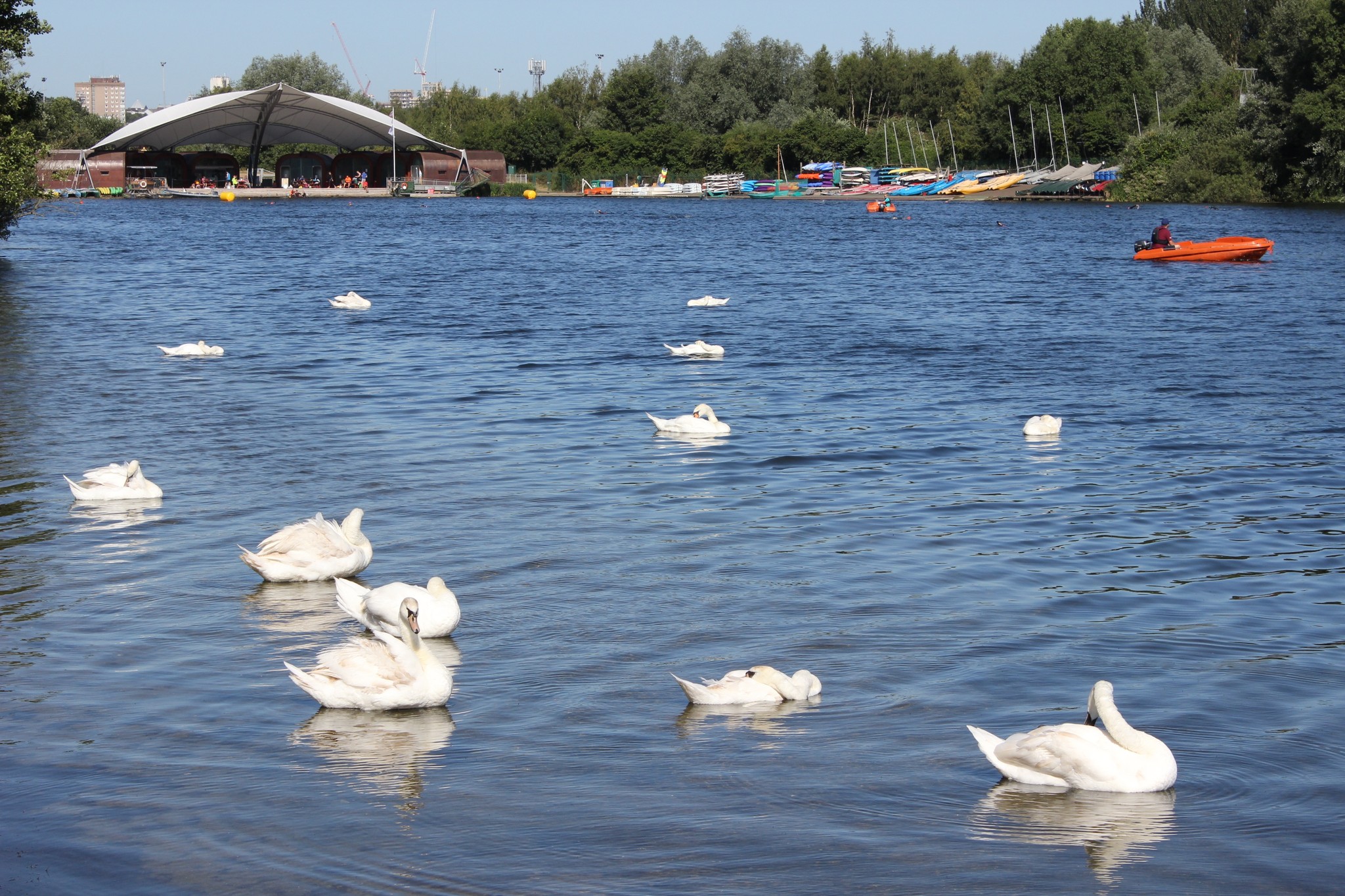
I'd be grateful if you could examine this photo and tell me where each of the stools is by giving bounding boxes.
[345,183,351,188]
[354,184,359,188]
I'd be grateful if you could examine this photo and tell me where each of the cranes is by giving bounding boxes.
[414,8,437,90]
[330,21,375,99]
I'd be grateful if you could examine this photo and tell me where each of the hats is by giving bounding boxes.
[1161,219,1169,225]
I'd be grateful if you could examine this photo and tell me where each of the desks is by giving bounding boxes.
[304,180,313,183]
[238,180,245,184]
[351,180,354,181]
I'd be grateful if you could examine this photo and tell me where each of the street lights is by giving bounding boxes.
[40,77,49,103]
[494,68,504,94]
[160,61,167,109]
[594,54,605,74]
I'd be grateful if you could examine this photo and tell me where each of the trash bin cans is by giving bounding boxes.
[598,179,613,188]
[591,180,600,189]
[262,177,273,186]
[508,165,516,175]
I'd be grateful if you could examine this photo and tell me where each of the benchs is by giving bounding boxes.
[199,179,211,187]
[235,183,248,188]
[298,183,316,188]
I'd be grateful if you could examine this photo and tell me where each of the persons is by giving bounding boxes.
[231,175,240,189]
[327,172,342,188]
[243,175,251,189]
[273,181,277,188]
[224,171,231,188]
[302,191,306,197]
[342,170,369,193]
[290,188,301,197]
[300,175,307,184]
[880,194,891,207]
[1151,219,1181,251]
[202,176,207,189]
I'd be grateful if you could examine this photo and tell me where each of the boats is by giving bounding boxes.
[866,201,896,213]
[1016,160,1118,197]
[583,186,613,195]
[124,165,173,200]
[51,187,124,198]
[795,160,1026,196]
[701,171,800,200]
[1132,236,1275,262]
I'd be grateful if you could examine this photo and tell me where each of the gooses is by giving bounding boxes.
[670,664,823,704]
[283,596,459,710]
[964,680,1179,791]
[62,459,164,499]
[687,296,730,307]
[236,507,373,582]
[645,403,732,434]
[663,340,725,354]
[332,575,461,639]
[289,703,457,818]
[1023,415,1062,436]
[158,340,225,356]
[328,291,373,307]
[966,772,1177,896]
